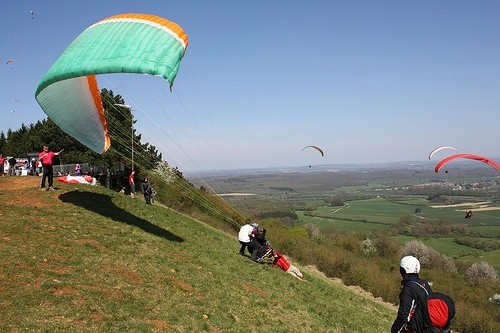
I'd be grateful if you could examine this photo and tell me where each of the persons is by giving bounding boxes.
[391,255,431,333]
[239,219,260,261]
[0,144,157,205]
[465,211,473,219]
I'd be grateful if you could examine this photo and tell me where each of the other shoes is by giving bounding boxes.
[240,250,244,255]
[50,186,56,190]
[41,187,46,191]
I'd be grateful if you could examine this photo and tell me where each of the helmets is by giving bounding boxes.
[399,255,421,274]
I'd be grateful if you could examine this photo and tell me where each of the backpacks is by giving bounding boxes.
[426,291,455,328]
